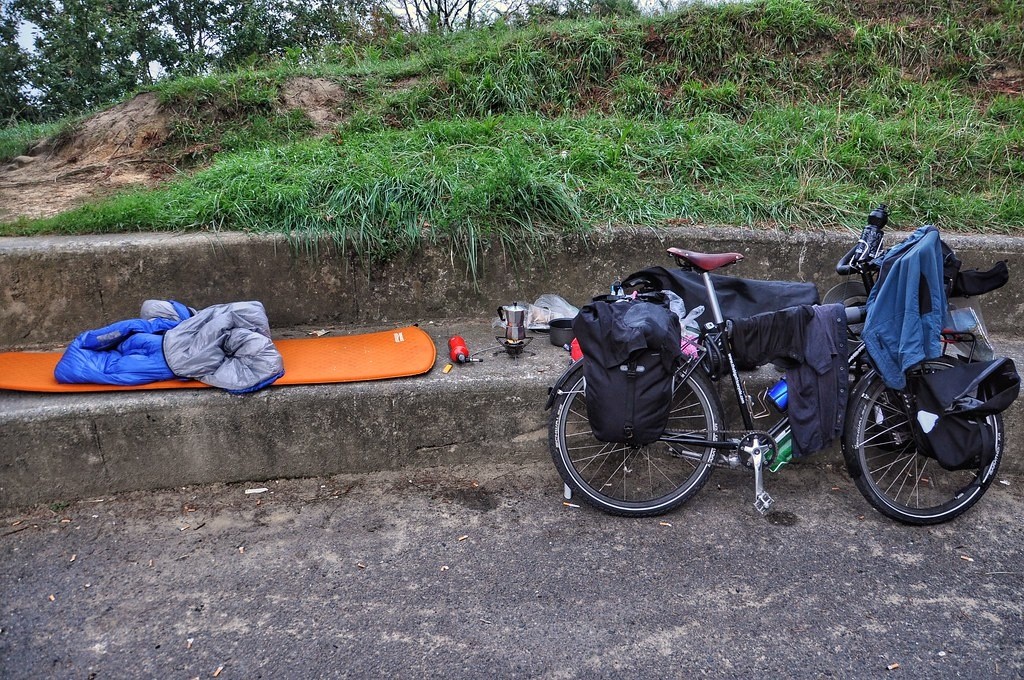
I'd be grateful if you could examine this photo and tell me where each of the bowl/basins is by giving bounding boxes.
[549,318,576,347]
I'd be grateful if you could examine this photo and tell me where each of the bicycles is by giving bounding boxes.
[544,207,1003,523]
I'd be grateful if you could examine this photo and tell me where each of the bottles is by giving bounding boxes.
[766,434,793,472]
[851,204,893,263]
[766,376,787,412]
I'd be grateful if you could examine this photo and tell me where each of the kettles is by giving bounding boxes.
[498,301,526,340]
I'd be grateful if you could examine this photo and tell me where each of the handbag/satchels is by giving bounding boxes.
[915,357,1020,471]
[572,298,680,448]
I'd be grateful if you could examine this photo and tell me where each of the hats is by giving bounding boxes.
[822,281,872,343]
